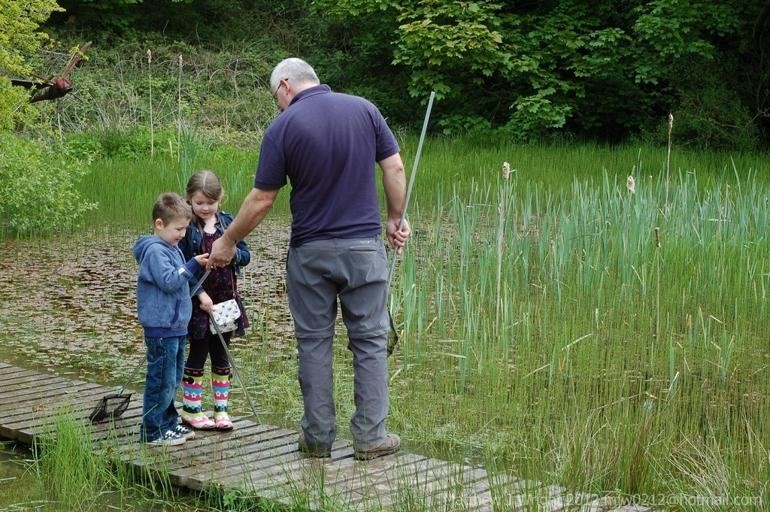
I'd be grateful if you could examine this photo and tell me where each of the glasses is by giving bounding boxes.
[271,78,289,103]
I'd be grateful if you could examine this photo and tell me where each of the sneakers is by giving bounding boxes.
[141,424,195,446]
[354,433,401,459]
[298,432,331,458]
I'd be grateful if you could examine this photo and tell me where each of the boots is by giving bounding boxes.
[211,366,232,429]
[182,367,215,430]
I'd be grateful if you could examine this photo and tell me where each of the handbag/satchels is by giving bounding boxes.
[209,299,244,336]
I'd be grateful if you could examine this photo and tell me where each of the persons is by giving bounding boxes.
[131,192,210,446]
[178,170,250,430]
[206,57,411,460]
